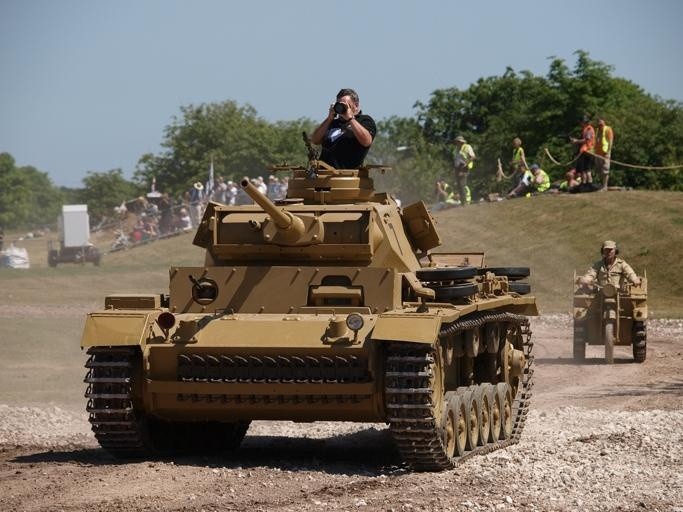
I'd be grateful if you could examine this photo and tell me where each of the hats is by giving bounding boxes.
[193,180,206,191]
[453,135,466,143]
[601,239,619,253]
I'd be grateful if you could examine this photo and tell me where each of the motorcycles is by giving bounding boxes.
[572,270,649,364]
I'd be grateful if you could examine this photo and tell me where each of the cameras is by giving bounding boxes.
[334,102,347,114]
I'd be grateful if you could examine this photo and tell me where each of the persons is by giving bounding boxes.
[581,240,639,293]
[427,114,613,212]
[109,174,290,252]
[311,88,376,168]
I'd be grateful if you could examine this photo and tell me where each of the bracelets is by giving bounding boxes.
[347,115,355,122]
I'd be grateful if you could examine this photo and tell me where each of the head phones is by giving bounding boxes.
[601,241,619,255]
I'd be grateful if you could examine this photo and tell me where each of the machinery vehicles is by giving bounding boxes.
[81,131,540,472]
